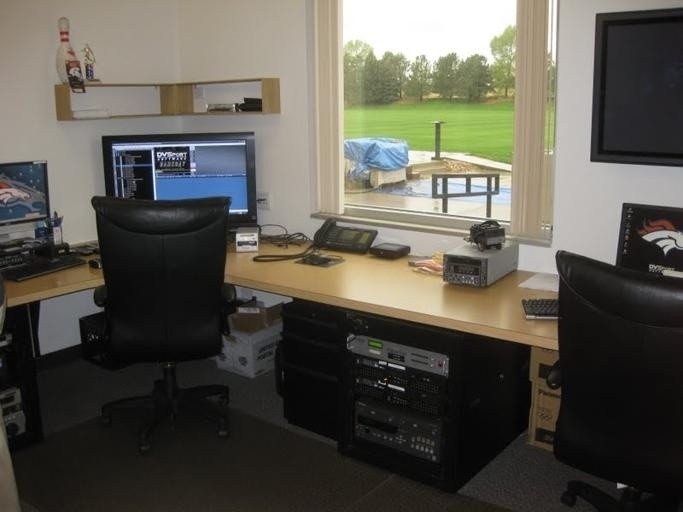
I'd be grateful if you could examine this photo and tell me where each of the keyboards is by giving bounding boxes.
[3,255,88,282]
[521,298,559,320]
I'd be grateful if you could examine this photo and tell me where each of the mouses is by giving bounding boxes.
[88,258,102,269]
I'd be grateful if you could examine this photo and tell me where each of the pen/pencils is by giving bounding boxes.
[48,211,63,227]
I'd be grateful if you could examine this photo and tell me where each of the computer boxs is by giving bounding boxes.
[0,304,43,452]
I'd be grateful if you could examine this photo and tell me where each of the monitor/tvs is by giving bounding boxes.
[1,159,51,242]
[101,132,257,228]
[615,202,683,278]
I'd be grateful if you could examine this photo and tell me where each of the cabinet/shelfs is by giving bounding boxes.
[53,76,281,122]
[1,240,563,456]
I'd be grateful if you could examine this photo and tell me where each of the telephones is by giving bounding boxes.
[313,219,377,253]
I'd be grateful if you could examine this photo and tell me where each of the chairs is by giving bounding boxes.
[553,250,682,512]
[79,197,237,454]
